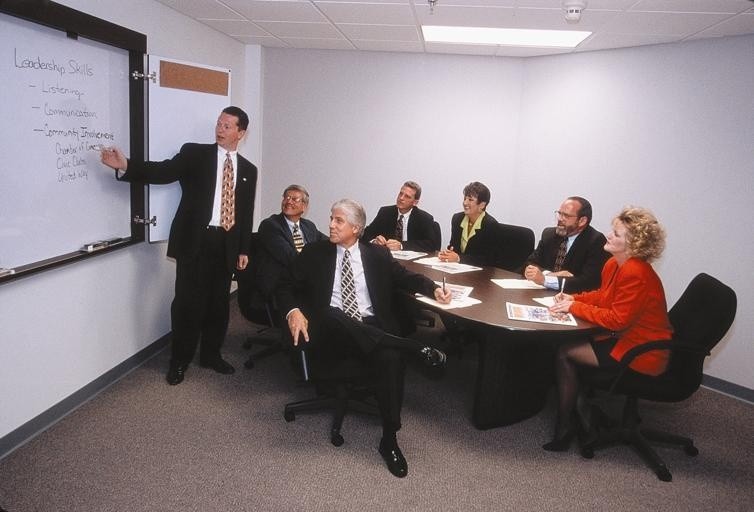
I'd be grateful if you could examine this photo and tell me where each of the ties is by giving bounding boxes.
[553,237,568,272]
[394,215,404,241]
[221,153,235,231]
[341,250,363,322]
[292,224,305,252]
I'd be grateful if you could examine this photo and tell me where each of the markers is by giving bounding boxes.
[90,145,116,152]
[104,237,124,246]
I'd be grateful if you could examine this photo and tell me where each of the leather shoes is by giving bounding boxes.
[166,364,187,385]
[420,345,446,368]
[200,358,235,374]
[379,438,408,477]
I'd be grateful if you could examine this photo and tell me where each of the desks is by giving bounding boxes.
[372,250,606,431]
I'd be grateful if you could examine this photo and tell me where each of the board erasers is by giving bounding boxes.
[79,241,108,252]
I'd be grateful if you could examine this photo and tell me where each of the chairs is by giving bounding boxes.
[480,219,534,274]
[572,270,737,479]
[237,234,314,376]
[282,302,408,454]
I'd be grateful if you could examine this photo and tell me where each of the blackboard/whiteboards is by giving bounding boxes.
[0,13,131,280]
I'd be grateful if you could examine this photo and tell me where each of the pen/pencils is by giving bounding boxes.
[442,276,446,296]
[559,277,566,301]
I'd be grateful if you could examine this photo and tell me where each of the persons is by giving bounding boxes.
[439,181,500,268]
[248,185,331,286]
[101,106,257,385]
[277,198,448,478]
[521,197,613,293]
[363,182,437,252]
[542,209,673,451]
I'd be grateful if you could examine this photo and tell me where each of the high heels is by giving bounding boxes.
[543,427,579,451]
